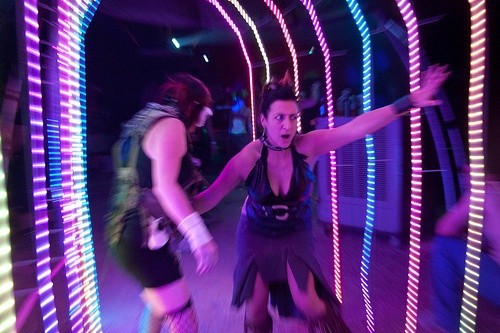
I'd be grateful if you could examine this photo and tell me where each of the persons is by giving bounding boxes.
[431,147,500,333]
[105,72,219,333]
[191,80,320,184]
[146,64,452,333]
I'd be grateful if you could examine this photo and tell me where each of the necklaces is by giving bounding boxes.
[260,134,295,151]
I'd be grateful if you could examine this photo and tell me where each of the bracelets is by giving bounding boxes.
[393,94,415,114]
